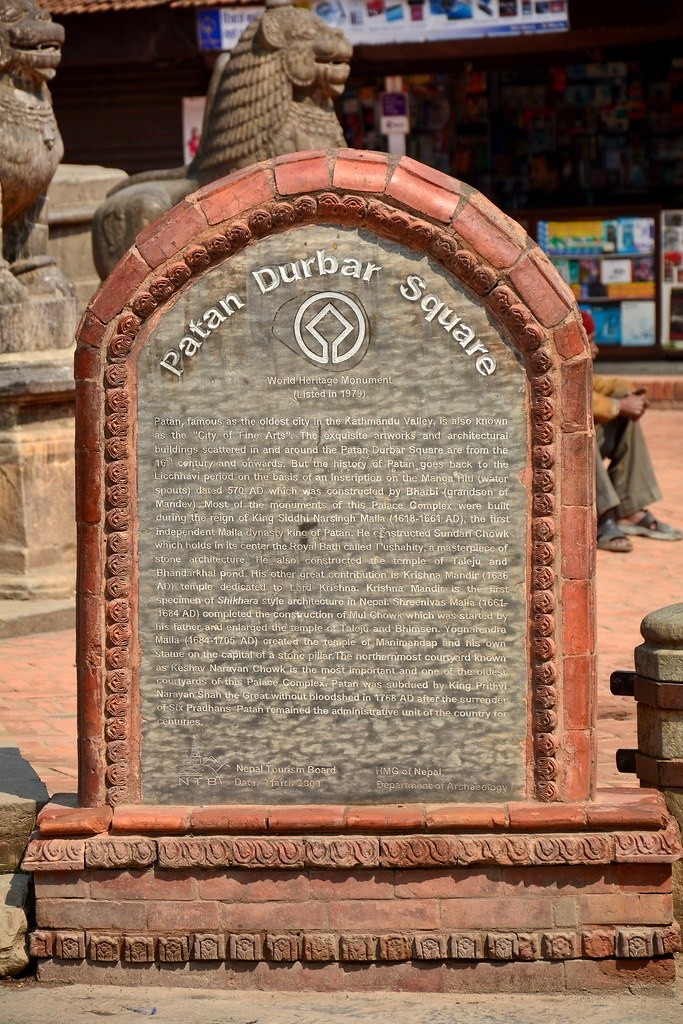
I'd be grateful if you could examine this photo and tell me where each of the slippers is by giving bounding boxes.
[597,518,634,552]
[617,511,683,541]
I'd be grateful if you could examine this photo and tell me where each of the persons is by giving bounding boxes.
[579,310,683,552]
